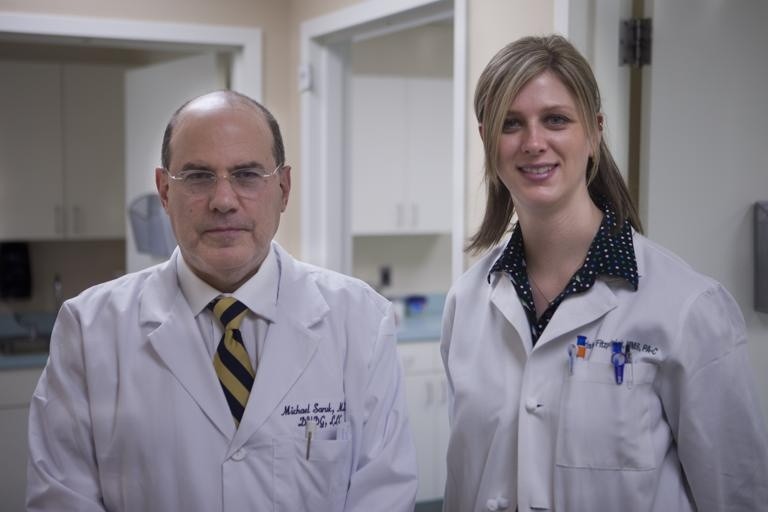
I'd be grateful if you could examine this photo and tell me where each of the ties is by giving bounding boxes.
[207,296,256,432]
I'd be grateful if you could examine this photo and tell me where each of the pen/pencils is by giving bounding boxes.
[625,344,639,363]
[303,418,316,462]
[609,341,627,386]
[565,344,578,379]
[575,334,587,357]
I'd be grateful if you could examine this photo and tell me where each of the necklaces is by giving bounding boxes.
[526,270,550,304]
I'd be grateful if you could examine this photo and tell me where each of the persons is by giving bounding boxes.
[440,34,768,512]
[27,88,420,512]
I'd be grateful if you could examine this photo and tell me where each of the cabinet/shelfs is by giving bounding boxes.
[0,369,44,512]
[0,59,143,242]
[398,341,450,503]
[348,75,453,237]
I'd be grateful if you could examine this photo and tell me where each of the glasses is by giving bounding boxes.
[161,161,286,201]
